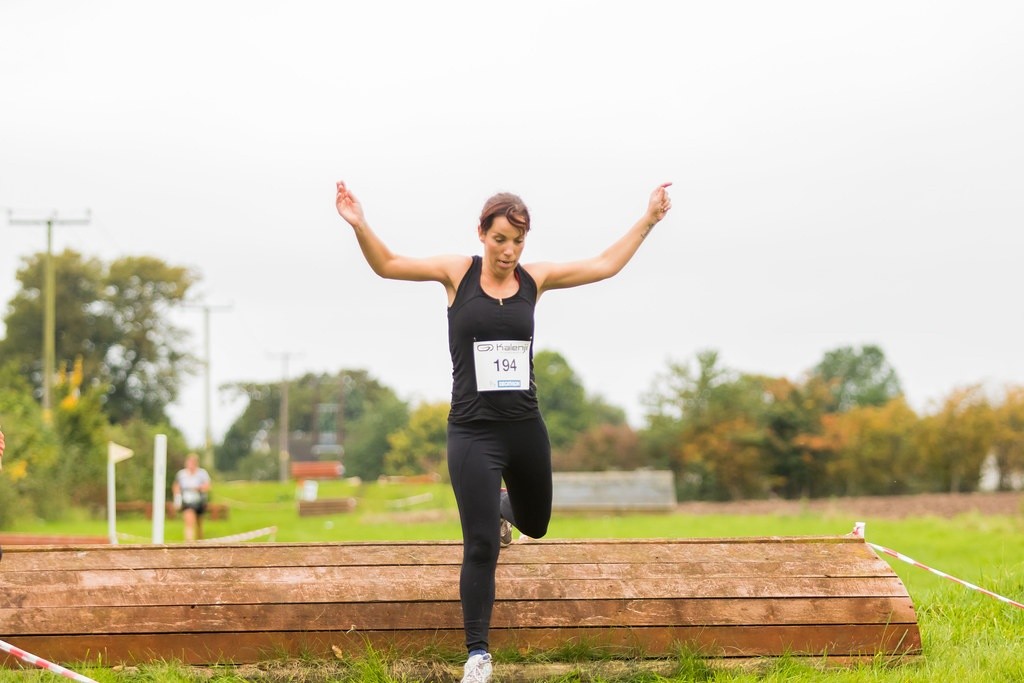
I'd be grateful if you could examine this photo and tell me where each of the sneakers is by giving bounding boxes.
[498,488,513,547]
[460,653,493,683]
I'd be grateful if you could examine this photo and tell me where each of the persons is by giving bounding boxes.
[335,183,671,683]
[172,453,211,542]
